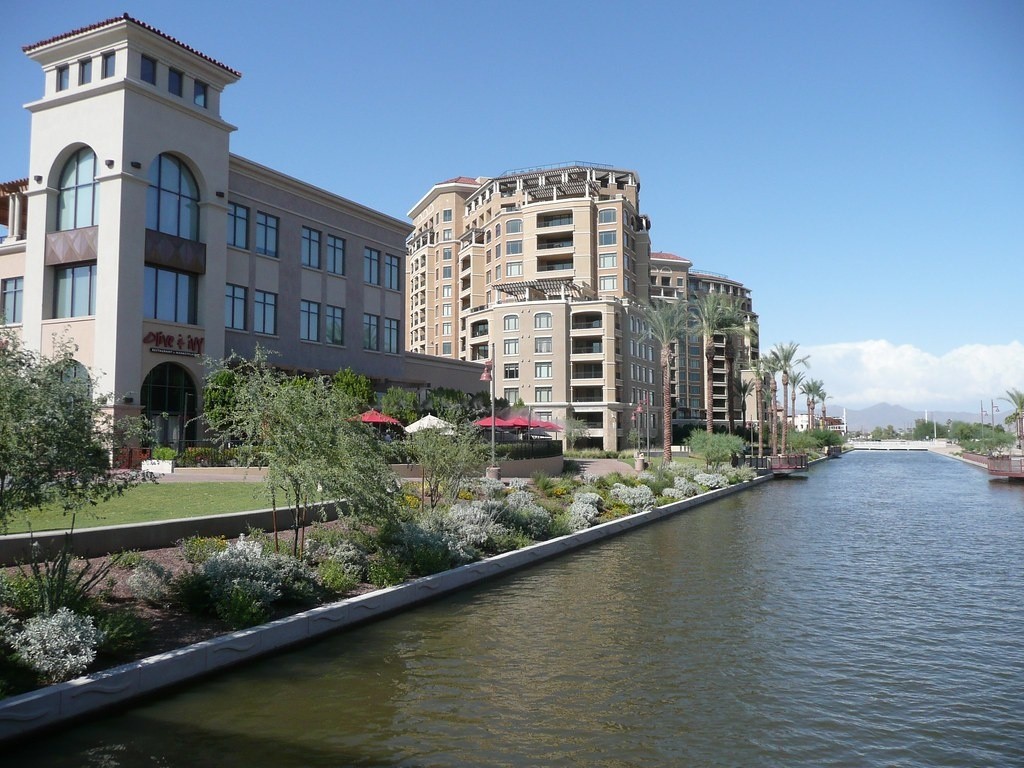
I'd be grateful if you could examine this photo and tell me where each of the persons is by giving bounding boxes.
[385,434,391,441]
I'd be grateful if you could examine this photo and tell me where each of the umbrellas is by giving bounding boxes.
[473,416,514,426]
[405,413,456,432]
[345,408,399,437]
[534,420,563,432]
[503,416,538,434]
[439,428,461,436]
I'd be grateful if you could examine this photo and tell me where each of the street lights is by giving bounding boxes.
[631,389,650,461]
[479,342,496,469]
[980,399,988,455]
[991,399,999,456]
